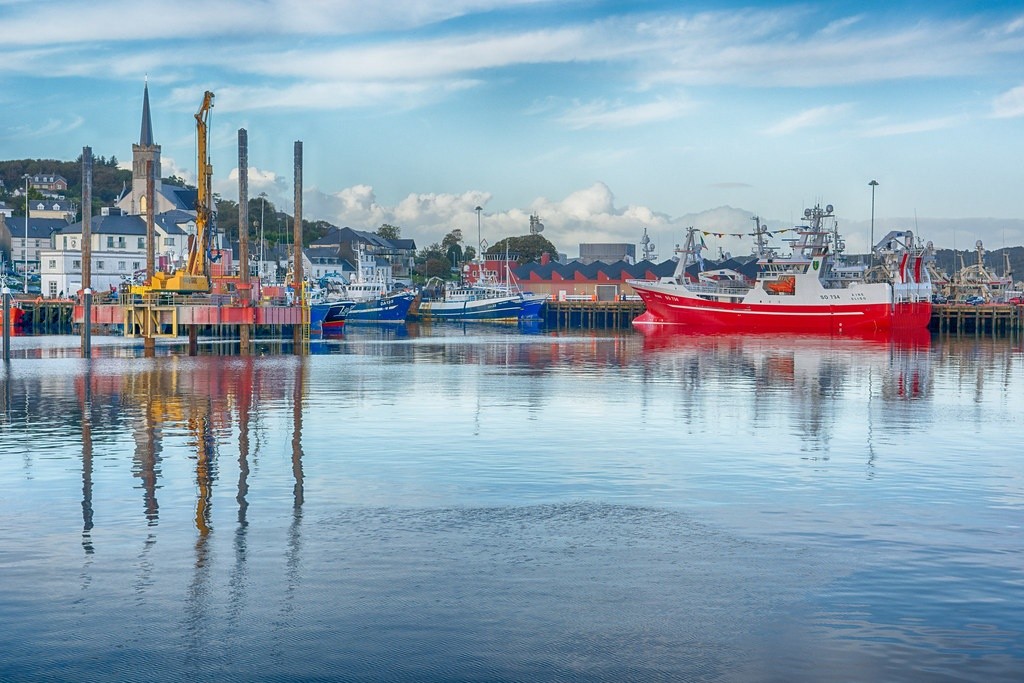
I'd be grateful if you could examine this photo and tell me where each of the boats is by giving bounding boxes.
[17,89,1024,364]
